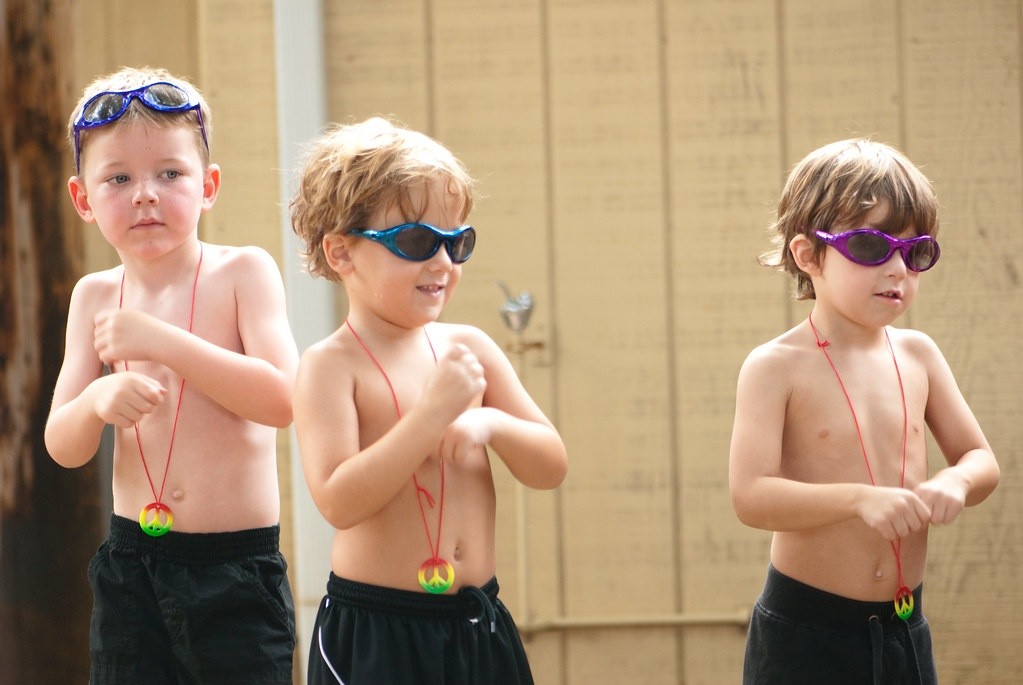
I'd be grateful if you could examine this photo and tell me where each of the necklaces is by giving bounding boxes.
[348,315,455,592]
[120,247,202,536]
[808,314,914,619]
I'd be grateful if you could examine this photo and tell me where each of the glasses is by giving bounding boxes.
[72,82,209,176]
[816,228,941,271]
[346,222,477,264]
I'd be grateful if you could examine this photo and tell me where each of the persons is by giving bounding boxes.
[730,139,1001,685]
[42,67,299,684]
[293,118,564,684]
